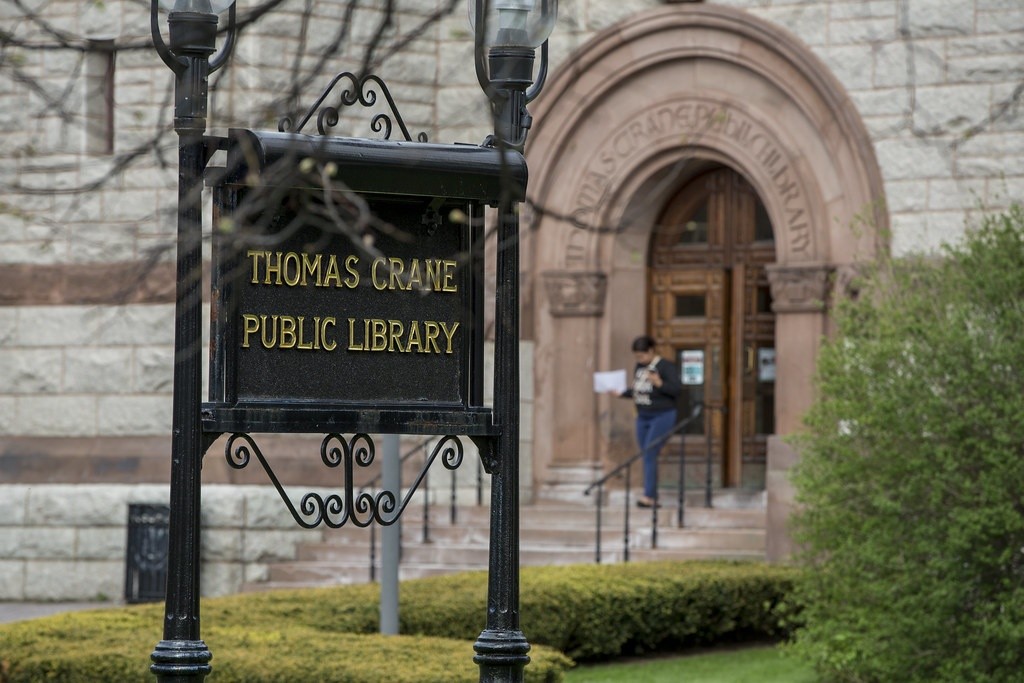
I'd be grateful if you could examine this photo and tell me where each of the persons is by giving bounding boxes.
[608,337,685,509]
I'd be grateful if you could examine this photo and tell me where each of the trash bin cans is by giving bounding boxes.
[125,502,170,603]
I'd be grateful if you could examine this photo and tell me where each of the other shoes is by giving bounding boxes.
[637,501,660,508]
